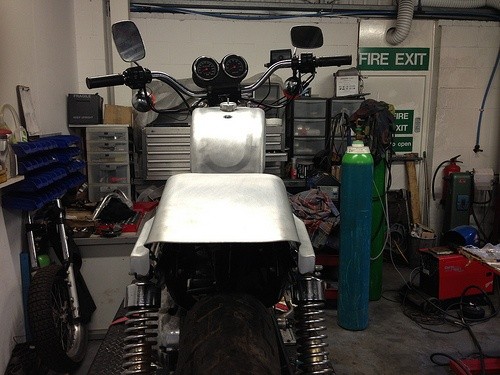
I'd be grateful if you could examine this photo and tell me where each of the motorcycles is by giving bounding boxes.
[83,20,354,375]
[27,199,98,375]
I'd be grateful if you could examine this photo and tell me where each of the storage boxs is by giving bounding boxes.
[334,75,359,97]
[68,93,133,126]
[289,98,364,162]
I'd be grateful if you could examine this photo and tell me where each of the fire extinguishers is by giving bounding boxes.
[430,153,464,210]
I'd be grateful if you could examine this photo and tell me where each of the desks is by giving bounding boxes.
[332,155,423,226]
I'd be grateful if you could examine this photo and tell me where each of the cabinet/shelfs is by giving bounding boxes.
[85,127,132,202]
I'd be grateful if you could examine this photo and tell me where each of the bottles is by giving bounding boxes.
[15,126,27,142]
